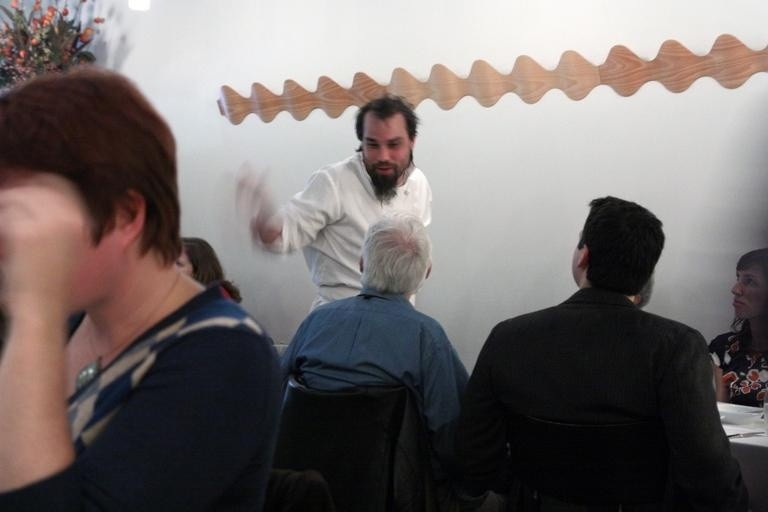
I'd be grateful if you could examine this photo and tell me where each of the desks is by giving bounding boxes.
[714,400,768,512]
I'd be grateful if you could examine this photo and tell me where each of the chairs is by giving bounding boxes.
[500,408,648,512]
[259,370,429,511]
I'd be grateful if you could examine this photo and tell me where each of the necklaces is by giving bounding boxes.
[74,273,181,395]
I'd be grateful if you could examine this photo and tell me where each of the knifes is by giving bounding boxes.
[725,431,764,439]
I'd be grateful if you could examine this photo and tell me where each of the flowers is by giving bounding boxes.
[0,0,105,90]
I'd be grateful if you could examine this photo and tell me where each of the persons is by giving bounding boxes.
[708,249,768,408]
[0,67,282,512]
[634,274,654,308]
[457,196,749,512]
[251,96,432,311]
[278,217,470,509]
[172,239,243,304]
